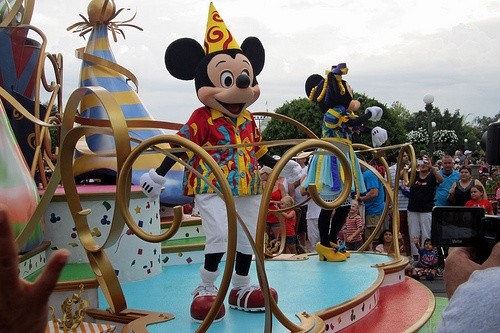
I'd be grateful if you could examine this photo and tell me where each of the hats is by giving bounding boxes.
[464,150,472,155]
[259,166,272,175]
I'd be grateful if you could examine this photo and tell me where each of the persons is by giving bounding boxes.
[434,241,500,333]
[355,148,500,279]
[255,151,331,252]
[0,207,70,333]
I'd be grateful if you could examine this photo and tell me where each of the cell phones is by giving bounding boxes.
[417,160,425,164]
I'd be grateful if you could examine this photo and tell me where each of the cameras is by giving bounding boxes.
[429,205,500,264]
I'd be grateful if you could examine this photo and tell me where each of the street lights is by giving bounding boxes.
[423,94,434,158]
[477,141,480,155]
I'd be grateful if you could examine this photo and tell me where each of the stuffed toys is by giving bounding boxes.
[140,1,303,324]
[304,63,388,261]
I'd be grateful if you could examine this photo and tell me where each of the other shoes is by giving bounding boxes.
[411,273,419,280]
[426,273,433,280]
